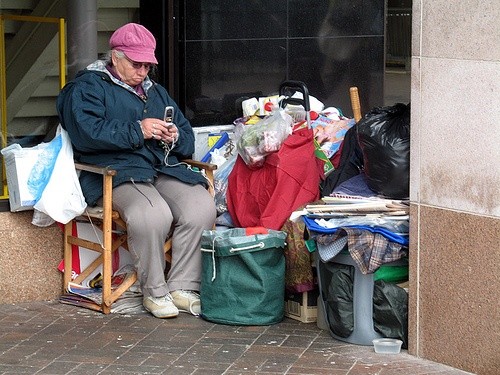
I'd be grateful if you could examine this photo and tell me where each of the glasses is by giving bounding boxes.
[124,57,158,70]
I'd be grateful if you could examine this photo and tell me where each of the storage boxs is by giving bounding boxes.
[1,141,62,213]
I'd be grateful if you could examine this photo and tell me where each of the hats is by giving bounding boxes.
[109,22,159,64]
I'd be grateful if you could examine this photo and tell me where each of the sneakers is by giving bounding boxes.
[143,293,179,317]
[171,290,202,315]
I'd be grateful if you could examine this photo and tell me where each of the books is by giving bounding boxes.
[303,195,409,229]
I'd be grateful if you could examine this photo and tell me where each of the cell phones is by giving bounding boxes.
[164,106,174,131]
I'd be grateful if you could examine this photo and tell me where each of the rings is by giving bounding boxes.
[153,123,156,128]
[151,135,155,139]
[152,129,156,134]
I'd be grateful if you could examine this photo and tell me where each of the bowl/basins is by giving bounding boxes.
[372,338,403,353]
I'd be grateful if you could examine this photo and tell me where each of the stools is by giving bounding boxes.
[64,155,218,315]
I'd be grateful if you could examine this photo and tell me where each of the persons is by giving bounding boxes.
[56,23,217,319]
